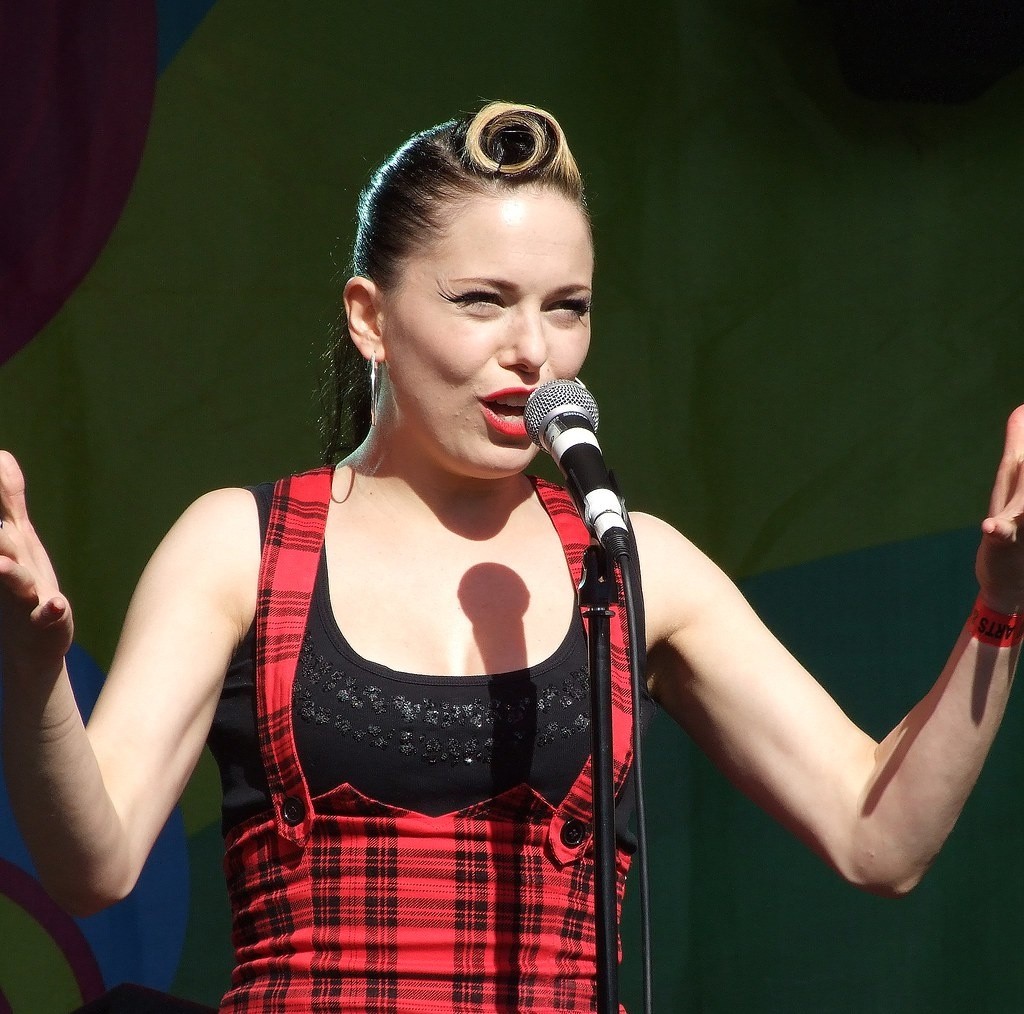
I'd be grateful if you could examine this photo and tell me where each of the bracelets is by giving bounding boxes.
[968,596,1024,648]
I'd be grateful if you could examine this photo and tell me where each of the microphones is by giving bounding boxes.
[523,379,630,559]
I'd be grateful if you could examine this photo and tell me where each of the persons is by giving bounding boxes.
[0,99,1024,1014]
